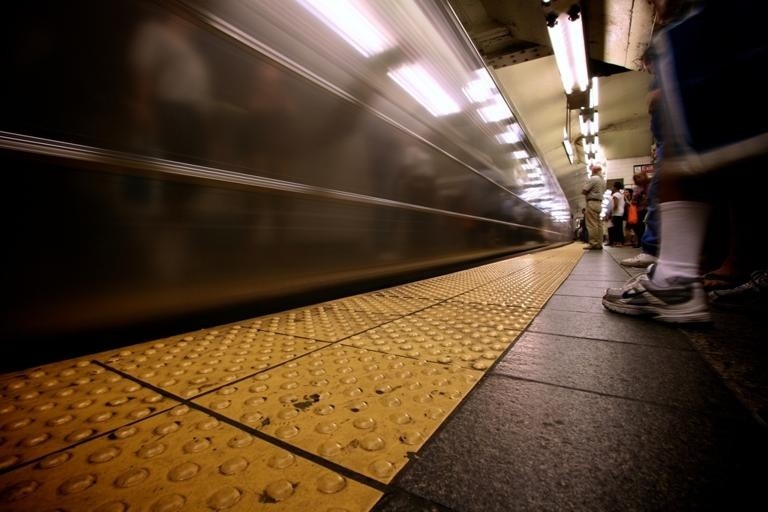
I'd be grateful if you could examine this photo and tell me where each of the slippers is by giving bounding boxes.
[703,273,751,292]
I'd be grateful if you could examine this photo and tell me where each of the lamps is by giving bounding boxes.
[545,6,601,184]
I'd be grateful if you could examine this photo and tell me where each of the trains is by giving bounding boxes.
[0,2,576,375]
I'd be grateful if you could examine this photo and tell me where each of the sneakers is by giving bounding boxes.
[601,264,711,325]
[620,252,658,267]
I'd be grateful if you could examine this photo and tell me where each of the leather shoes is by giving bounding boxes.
[583,245,602,250]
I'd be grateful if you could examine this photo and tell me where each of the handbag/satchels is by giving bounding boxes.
[628,205,638,225]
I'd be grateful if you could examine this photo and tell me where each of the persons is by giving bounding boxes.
[579,165,659,269]
[602,0,768,324]
